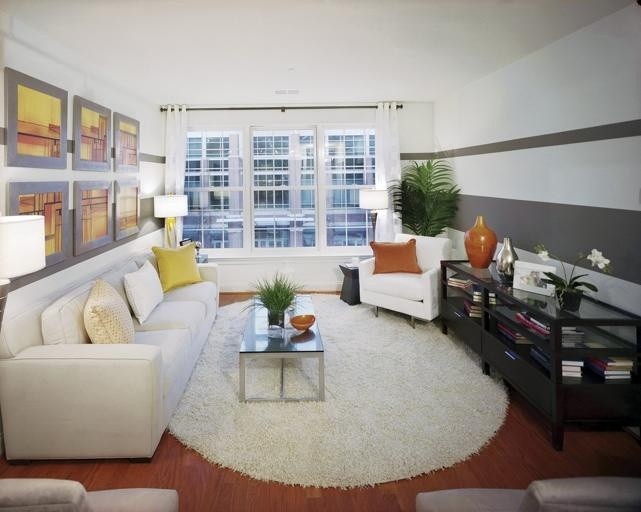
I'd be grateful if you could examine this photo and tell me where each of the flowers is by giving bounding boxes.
[532,242,612,301]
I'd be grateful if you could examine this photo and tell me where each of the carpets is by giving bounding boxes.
[165,292,510,490]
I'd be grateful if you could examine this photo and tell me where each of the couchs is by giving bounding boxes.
[359,235,450,328]
[413,477,640,511]
[0,256,219,464]
[0,478,177,512]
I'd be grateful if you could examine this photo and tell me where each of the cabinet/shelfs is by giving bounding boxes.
[481,284,640,450]
[440,259,511,357]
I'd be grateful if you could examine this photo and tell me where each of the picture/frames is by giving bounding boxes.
[2,67,67,170]
[7,181,72,264]
[113,179,141,239]
[72,94,111,173]
[74,181,112,257]
[512,260,557,298]
[111,111,141,173]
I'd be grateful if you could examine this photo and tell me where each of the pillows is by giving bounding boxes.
[151,242,202,292]
[370,239,422,275]
[121,260,164,325]
[81,276,134,344]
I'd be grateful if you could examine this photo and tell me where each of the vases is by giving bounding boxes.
[495,237,519,284]
[464,214,498,269]
[554,288,583,311]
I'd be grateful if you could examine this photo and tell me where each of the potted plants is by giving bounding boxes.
[239,271,306,336]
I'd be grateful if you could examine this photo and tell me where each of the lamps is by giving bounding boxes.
[214,186,229,198]
[152,194,189,248]
[358,188,390,248]
[0,216,46,284]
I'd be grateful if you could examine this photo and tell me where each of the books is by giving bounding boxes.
[448,272,634,380]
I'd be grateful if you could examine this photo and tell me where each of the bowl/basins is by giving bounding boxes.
[290,314,316,332]
